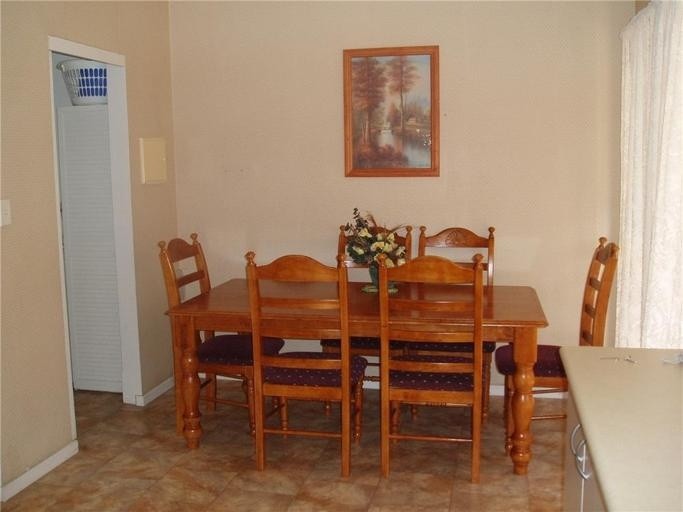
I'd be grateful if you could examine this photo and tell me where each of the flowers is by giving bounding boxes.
[344,212,410,267]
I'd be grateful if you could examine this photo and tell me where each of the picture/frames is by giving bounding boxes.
[340,43,442,183]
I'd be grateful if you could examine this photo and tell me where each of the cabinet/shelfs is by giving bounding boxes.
[555,338,682,511]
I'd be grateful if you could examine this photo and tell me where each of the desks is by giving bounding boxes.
[163,278,546,475]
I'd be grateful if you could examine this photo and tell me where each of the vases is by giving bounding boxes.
[365,263,394,288]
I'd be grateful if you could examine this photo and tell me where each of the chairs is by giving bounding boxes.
[153,230,284,439]
[494,234,617,457]
[318,222,410,417]
[409,217,495,423]
[242,249,367,478]
[371,251,488,486]
